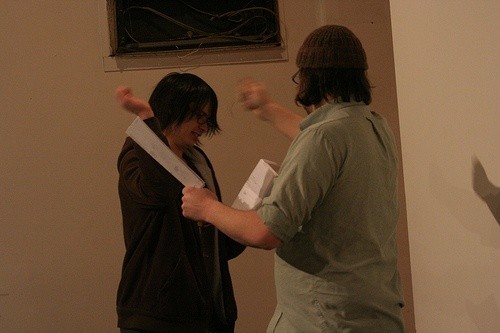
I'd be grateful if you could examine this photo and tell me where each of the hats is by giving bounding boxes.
[296,25,368,68]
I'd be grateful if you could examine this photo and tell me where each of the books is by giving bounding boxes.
[231,160,280,212]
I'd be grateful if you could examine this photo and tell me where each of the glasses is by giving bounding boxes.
[292,69,301,84]
[190,108,214,127]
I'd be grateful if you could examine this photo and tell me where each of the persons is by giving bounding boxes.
[116,72,246,333]
[180,25,405,333]
